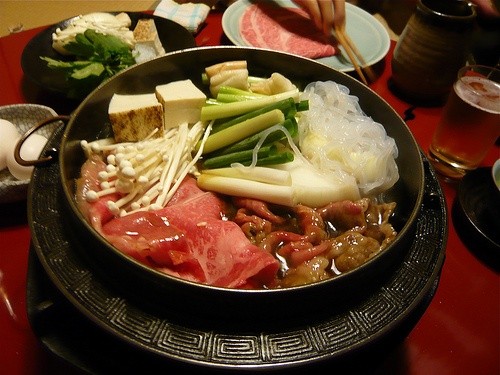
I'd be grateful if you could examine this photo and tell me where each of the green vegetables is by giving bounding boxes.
[39,27,137,80]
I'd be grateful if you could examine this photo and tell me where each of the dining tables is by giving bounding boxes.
[1,9,500,375]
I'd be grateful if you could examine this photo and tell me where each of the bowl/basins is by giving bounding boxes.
[0,104,61,194]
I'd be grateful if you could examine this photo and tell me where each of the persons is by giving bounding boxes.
[291,0,346,36]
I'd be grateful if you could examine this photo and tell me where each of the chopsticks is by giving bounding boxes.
[337,27,377,88]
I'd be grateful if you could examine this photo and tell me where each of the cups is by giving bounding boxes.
[390,1,481,106]
[427,64,499,184]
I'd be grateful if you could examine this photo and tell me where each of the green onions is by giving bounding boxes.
[192,86,309,206]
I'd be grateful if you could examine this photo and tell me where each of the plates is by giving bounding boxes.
[18,9,200,112]
[15,41,427,294]
[25,121,448,370]
[222,0,390,70]
[25,237,442,375]
